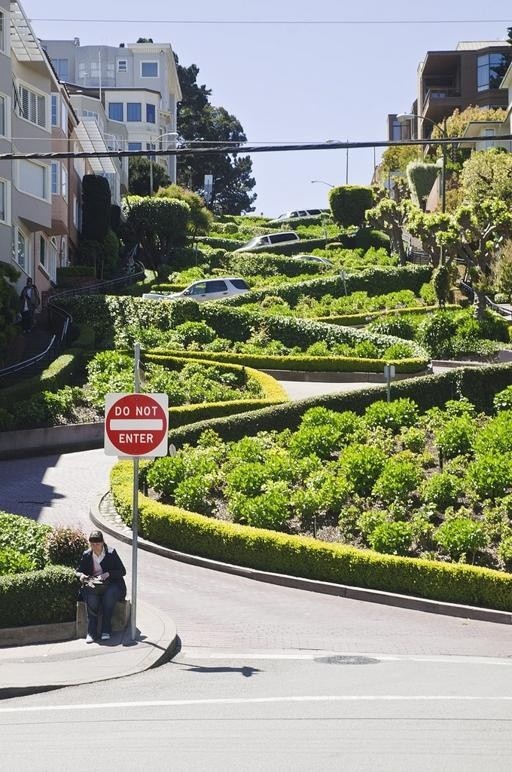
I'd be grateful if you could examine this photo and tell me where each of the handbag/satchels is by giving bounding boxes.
[84,575,109,595]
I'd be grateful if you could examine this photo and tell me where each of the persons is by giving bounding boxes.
[22,278,40,328]
[17,280,41,335]
[73,530,127,643]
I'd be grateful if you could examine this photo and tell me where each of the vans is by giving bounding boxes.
[237,232,300,251]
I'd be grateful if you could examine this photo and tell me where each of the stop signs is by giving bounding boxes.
[104,393,169,457]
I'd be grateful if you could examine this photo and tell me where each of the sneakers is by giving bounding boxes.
[85,633,96,642]
[101,633,112,640]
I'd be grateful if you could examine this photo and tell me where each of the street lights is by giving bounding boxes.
[396,113,447,266]
[311,139,349,188]
[149,132,178,194]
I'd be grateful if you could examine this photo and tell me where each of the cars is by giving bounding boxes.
[271,209,323,223]
[164,278,252,301]
[294,255,334,265]
